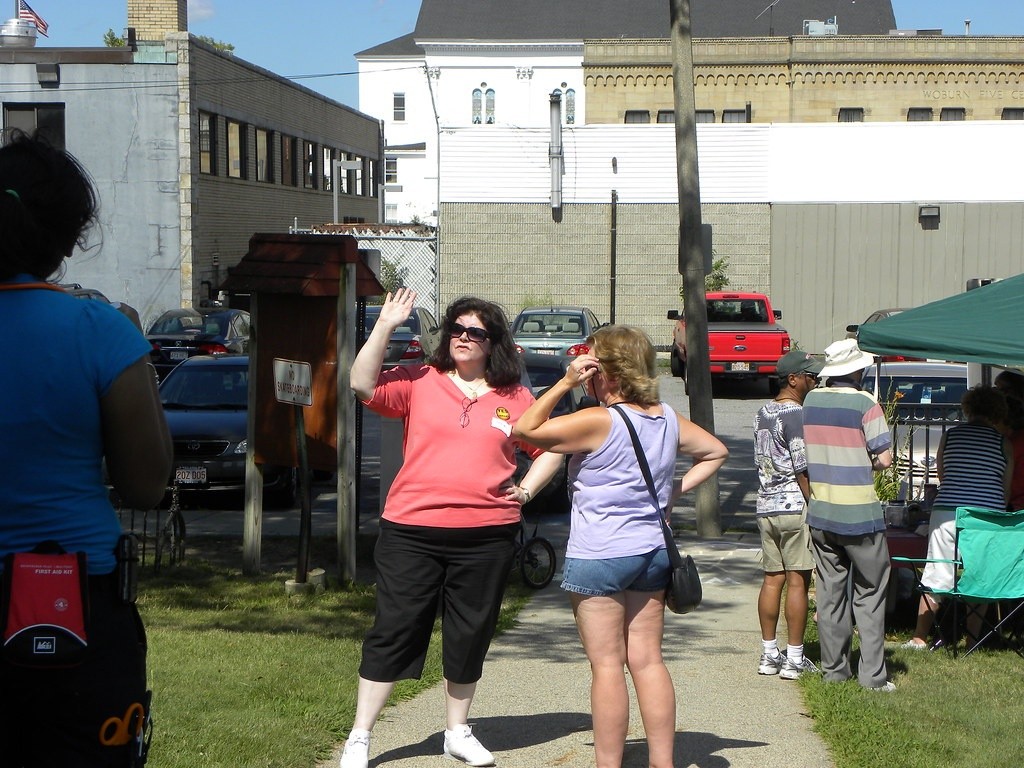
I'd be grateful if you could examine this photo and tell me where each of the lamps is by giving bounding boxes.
[918,204,940,218]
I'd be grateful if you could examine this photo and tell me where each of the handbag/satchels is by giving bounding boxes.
[666,555,702,614]
[4,540,89,669]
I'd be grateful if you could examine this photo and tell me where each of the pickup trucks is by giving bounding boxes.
[667,291,790,396]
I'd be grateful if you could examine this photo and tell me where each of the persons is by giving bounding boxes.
[803,340,895,692]
[0,135,175,768]
[901,368,1024,650]
[753,351,824,680]
[513,327,729,768]
[338,287,567,768]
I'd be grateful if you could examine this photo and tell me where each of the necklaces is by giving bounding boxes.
[455,370,486,398]
[775,397,802,403]
[607,401,630,408]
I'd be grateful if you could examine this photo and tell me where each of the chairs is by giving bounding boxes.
[366,315,416,334]
[891,506,1023,658]
[168,317,221,335]
[740,301,757,321]
[521,318,581,335]
[187,371,224,406]
[536,387,568,410]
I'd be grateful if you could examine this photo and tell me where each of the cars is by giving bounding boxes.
[860,362,968,502]
[364,305,442,374]
[846,307,966,362]
[148,306,251,387]
[509,305,614,357]
[514,349,599,515]
[156,355,289,510]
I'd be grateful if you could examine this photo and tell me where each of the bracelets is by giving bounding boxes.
[146,362,159,381]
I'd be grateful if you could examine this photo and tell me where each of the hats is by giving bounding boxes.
[776,351,826,379]
[817,338,874,377]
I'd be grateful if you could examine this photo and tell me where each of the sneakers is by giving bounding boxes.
[864,681,897,694]
[779,653,824,680]
[757,646,786,675]
[340,728,372,768]
[900,640,927,651]
[443,723,495,766]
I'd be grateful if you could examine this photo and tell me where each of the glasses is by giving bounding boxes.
[794,372,817,380]
[460,397,477,428]
[450,323,503,342]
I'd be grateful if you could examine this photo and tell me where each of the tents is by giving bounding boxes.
[857,274,1024,399]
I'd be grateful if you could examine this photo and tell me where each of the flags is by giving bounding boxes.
[20,0,49,37]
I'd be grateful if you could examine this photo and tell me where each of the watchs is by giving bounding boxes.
[520,487,530,504]
